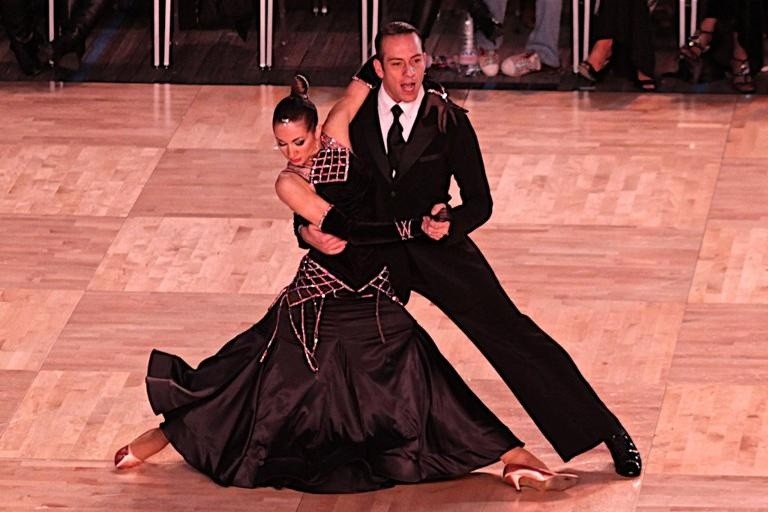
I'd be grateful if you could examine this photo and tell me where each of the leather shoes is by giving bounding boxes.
[605,427,644,478]
[634,70,657,93]
[113,426,166,471]
[576,58,611,83]
[728,54,755,95]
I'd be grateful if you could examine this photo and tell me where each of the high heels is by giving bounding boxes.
[675,27,714,63]
[500,461,581,493]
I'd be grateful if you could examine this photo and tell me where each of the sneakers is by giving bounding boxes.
[499,49,544,78]
[478,51,502,80]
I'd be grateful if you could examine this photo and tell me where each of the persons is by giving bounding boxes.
[290,18,643,477]
[574,0,662,94]
[675,0,767,94]
[473,0,566,79]
[109,53,584,494]
[4,1,107,84]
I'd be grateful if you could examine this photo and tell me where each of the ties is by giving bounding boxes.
[385,103,409,174]
